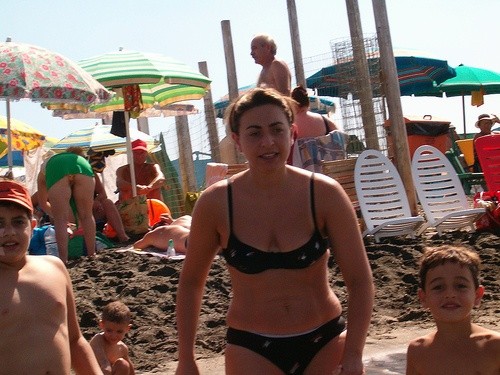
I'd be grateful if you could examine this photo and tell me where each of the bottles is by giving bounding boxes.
[167,240,175,257]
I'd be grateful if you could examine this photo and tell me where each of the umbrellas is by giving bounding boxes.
[0,38,211,198]
[304,49,500,138]
[213,85,335,118]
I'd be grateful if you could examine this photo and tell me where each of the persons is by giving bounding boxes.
[0,140,197,375]
[177,88,374,375]
[472,114,500,193]
[252,37,343,170]
[405,245,500,375]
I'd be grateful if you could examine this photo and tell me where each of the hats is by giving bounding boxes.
[475,114,496,127]
[0,179,33,214]
[132,139,148,152]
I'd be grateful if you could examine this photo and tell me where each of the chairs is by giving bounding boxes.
[206,130,500,243]
